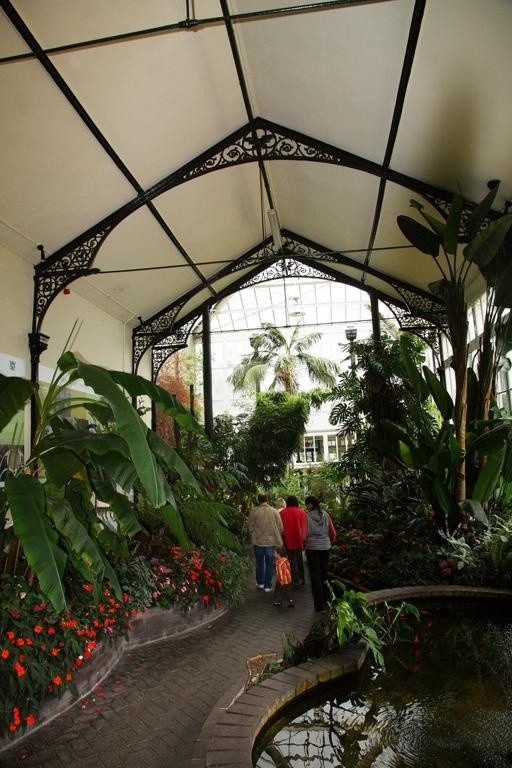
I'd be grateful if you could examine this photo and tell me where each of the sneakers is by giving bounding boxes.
[255,583,272,592]
[274,602,295,608]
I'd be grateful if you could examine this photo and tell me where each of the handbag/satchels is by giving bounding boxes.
[329,520,336,544]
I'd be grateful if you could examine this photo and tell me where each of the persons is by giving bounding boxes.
[248,493,336,611]
